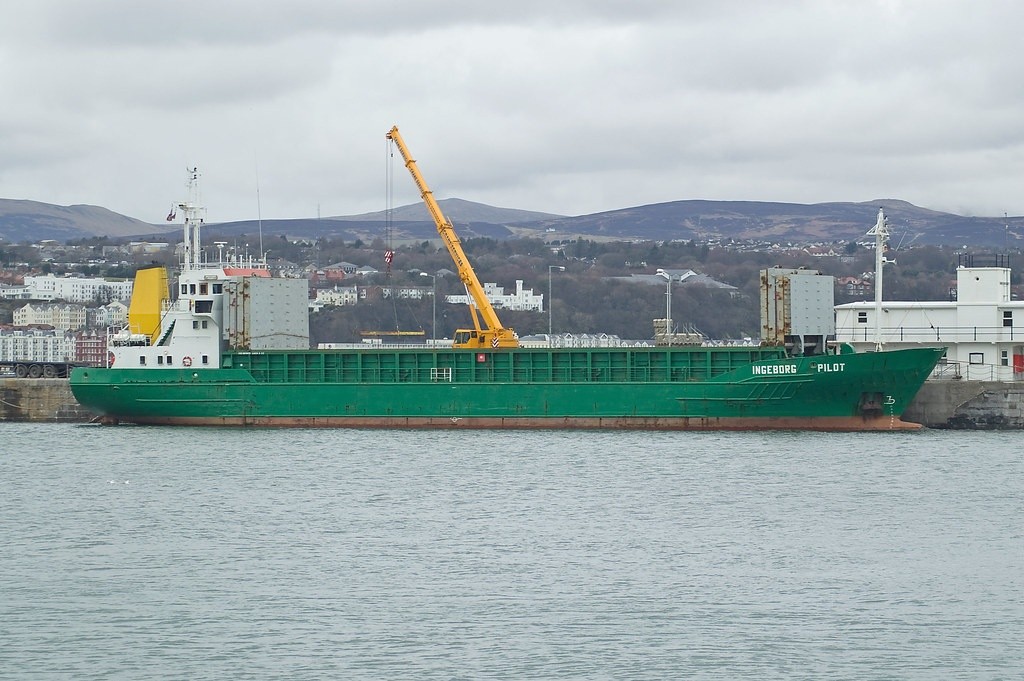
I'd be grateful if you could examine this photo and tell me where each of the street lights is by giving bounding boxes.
[549,266,566,335]
[657,269,671,347]
[420,272,436,349]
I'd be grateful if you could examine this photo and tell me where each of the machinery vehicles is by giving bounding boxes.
[379,127,521,348]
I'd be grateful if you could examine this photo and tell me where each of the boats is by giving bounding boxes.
[69,165,945,430]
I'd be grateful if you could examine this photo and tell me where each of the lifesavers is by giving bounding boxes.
[183,356,193,367]
